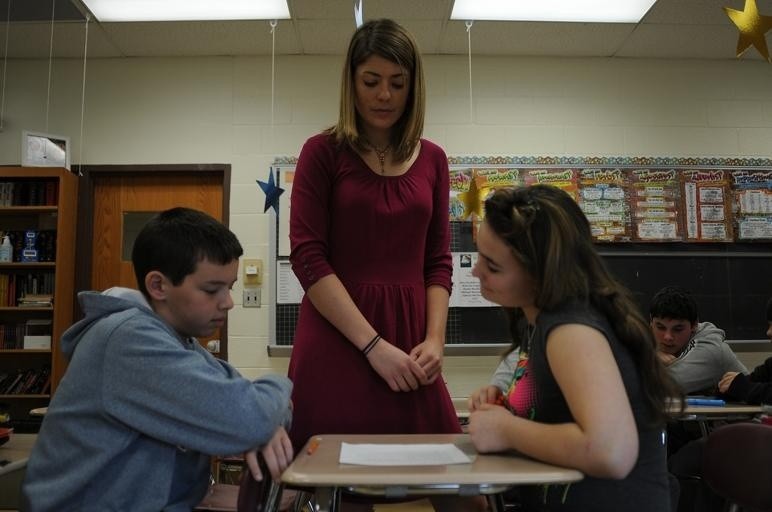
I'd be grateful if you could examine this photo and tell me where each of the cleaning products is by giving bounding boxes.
[0,236,14,263]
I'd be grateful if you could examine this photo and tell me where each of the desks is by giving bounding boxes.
[664,395,772,438]
[283,434,585,512]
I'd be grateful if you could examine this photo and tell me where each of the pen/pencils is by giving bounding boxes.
[685,398,726,407]
[307,438,322,455]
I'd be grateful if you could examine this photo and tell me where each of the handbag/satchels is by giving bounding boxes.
[668,418,712,512]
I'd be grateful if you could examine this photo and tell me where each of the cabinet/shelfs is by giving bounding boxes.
[0,164,80,426]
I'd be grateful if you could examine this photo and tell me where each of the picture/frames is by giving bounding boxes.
[21,129,71,172]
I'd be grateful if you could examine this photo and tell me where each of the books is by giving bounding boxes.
[1,272,55,394]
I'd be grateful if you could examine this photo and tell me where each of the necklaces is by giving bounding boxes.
[366,137,393,173]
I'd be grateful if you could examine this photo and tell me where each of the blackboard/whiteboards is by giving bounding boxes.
[266,156,772,357]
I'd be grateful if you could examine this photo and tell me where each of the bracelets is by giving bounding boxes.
[362,334,380,354]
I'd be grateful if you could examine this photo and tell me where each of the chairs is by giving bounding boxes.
[195,484,315,512]
[237,453,282,512]
[701,423,772,512]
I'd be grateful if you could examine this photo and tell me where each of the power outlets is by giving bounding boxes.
[243,288,261,307]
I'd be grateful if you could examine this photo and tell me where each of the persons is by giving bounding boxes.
[466,184,686,511]
[647,286,757,442]
[467,344,521,411]
[20,206,296,511]
[718,327,771,406]
[287,18,489,512]
